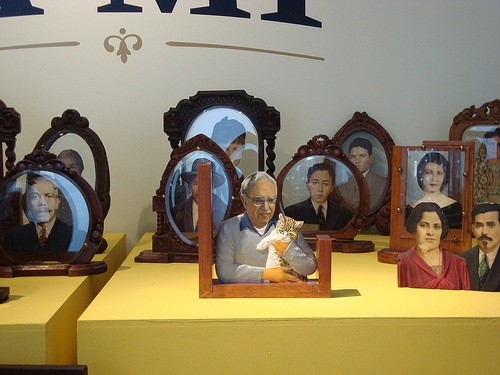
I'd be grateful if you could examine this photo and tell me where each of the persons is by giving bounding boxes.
[398,202,470,290]
[4,175,86,252]
[339,138,387,209]
[460,204,500,292]
[212,116,246,182]
[405,152,463,230]
[55,149,89,231]
[284,163,351,230]
[473,128,500,202]
[172,158,226,232]
[216,170,317,283]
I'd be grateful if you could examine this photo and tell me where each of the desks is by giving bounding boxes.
[77,232,500,375]
[0,233,127,366]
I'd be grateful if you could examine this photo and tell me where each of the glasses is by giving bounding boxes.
[246,194,277,206]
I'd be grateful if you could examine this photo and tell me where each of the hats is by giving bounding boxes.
[181,158,226,189]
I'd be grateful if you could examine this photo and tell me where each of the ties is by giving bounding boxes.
[39,226,47,248]
[318,205,326,224]
[478,254,489,278]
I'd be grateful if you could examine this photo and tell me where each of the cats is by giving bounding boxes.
[254,213,304,282]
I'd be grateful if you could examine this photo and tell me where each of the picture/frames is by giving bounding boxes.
[274,135,374,254]
[134,134,244,264]
[332,111,397,236]
[0,99,24,235]
[0,149,108,279]
[447,99,500,238]
[377,140,475,265]
[163,90,281,182]
[30,109,111,254]
